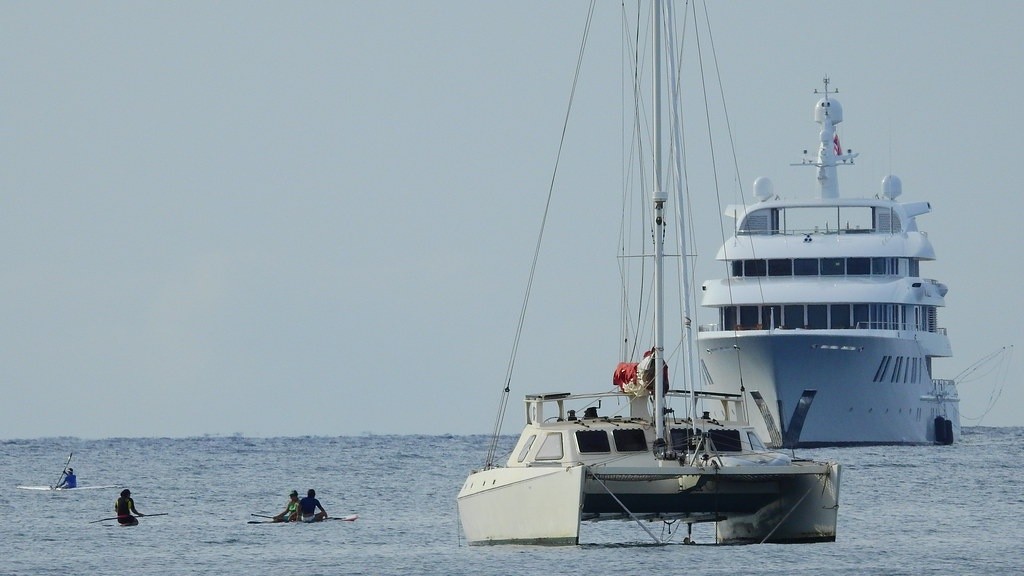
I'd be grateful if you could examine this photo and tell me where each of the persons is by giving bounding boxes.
[275,490,302,522]
[115,489,143,527]
[297,489,327,522]
[56,468,77,489]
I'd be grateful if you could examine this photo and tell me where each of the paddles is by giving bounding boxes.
[250,513,347,522]
[54,452,73,491]
[89,512,169,525]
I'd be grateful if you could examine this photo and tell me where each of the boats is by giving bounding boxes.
[694,73,964,446]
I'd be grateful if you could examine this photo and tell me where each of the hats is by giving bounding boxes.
[288,490,298,496]
[123,489,132,494]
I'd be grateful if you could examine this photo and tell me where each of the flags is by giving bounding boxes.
[834,135,842,155]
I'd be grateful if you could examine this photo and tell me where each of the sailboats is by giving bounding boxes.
[455,1,842,551]
